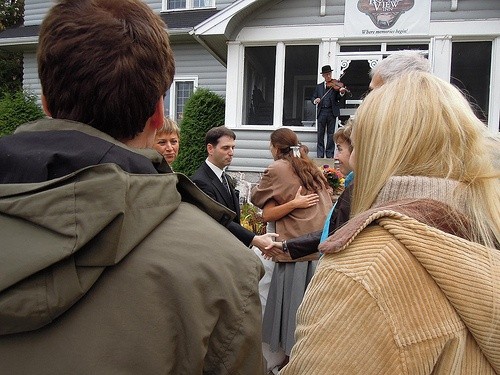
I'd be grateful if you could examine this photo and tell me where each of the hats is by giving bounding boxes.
[320,66,333,74]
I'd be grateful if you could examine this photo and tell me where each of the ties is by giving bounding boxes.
[221,172,230,195]
[326,83,328,89]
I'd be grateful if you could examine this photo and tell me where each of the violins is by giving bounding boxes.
[325,79,353,98]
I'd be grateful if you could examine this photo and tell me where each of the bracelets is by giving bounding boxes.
[282,240,287,253]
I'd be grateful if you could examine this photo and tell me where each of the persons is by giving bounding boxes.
[311,65,345,158]
[0,0,268,375]
[278,71,500,375]
[261,123,355,261]
[153,115,180,163]
[369,51,432,90]
[192,126,284,258]
[249,127,334,371]
